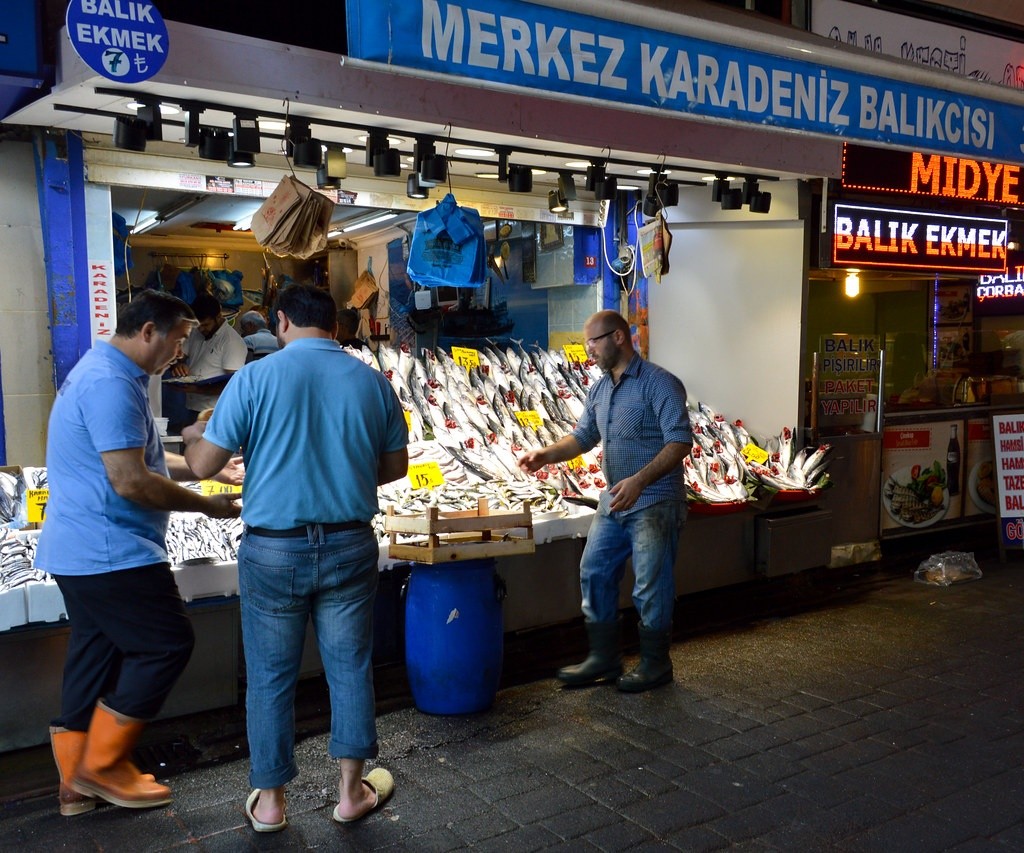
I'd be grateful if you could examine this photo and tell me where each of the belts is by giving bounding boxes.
[235,519,370,542]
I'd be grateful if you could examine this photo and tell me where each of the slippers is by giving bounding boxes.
[330,767,394,823]
[246,786,286,832]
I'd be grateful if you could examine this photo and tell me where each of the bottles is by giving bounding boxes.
[946,424,960,495]
[962,328,970,351]
[963,289,970,312]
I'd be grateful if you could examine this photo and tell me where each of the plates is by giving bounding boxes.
[943,309,966,321]
[882,466,949,528]
[968,460,997,515]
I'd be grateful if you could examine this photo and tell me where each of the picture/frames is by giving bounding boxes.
[540,222,564,251]
[483,220,497,242]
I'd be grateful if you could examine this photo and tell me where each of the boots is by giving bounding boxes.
[69,699,173,808]
[49,726,155,816]
[614,620,673,695]
[557,617,625,684]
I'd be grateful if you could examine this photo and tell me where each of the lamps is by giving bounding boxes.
[130,194,211,235]
[549,171,577,213]
[227,113,260,168]
[179,104,228,160]
[407,138,446,200]
[643,166,679,217]
[316,145,347,190]
[742,177,771,213]
[494,147,532,192]
[712,174,742,210]
[113,98,162,152]
[327,209,401,238]
[285,119,321,169]
[585,159,617,200]
[366,131,400,177]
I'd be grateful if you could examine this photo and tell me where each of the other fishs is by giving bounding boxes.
[0,338,835,591]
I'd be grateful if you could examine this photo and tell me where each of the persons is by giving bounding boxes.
[170,292,248,445]
[181,285,410,832]
[519,308,693,689]
[334,307,373,351]
[238,310,280,365]
[33,289,247,816]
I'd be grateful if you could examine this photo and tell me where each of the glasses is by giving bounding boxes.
[586,330,616,348]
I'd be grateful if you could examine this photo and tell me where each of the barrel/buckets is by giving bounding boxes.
[399,561,509,715]
